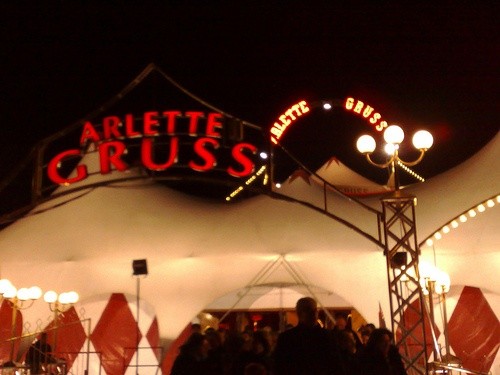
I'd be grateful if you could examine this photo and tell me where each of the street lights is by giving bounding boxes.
[0,279,42,367]
[357,125,434,375]
[46,291,79,352]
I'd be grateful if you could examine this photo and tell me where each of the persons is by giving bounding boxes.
[271,298,354,375]
[171,316,405,375]
[24,333,52,375]
[355,328,406,375]
